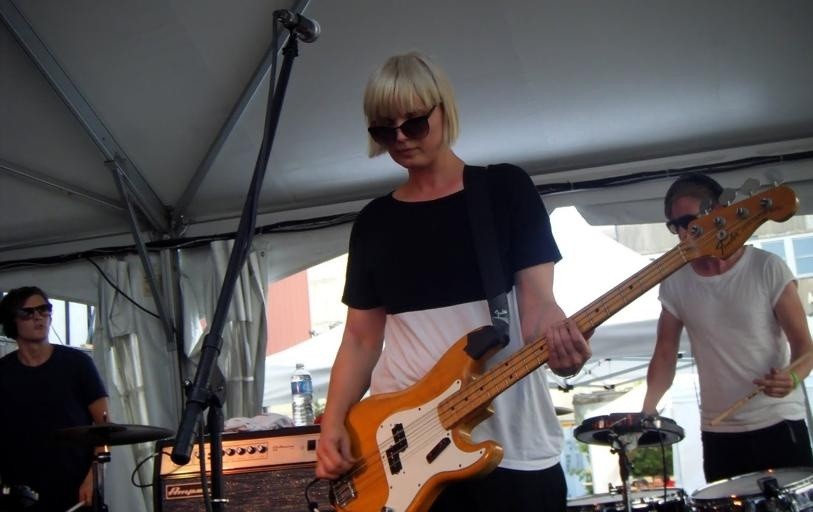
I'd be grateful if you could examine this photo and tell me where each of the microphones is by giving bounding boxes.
[281,9,322,42]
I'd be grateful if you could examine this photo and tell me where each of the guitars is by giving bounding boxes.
[325,177,799,512]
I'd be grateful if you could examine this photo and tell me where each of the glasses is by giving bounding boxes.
[666,214,703,235]
[17,303,54,320]
[367,106,438,147]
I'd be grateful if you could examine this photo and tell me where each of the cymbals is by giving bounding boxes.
[56,422,176,447]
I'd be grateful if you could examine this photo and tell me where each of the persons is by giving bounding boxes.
[312,51,592,511]
[638,171,812,486]
[0,286,111,512]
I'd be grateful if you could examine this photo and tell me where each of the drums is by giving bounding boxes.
[574,412,685,448]
[565,488,692,512]
[690,467,812,511]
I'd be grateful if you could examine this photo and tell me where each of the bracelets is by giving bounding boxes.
[788,369,799,390]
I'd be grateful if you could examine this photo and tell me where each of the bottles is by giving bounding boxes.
[290,363,315,427]
